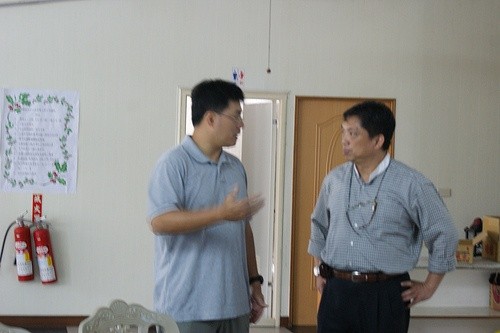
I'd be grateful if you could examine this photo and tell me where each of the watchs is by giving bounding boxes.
[313,266,320,276]
[249,275,263,284]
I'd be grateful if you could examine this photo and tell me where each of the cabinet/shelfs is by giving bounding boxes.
[409,257,500,318]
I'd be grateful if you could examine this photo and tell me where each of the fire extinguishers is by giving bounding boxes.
[12,209,35,282]
[29,215,57,285]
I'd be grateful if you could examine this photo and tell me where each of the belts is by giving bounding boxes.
[330,268,407,283]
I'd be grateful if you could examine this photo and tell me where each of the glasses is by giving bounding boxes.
[345,200,378,236]
[212,108,244,125]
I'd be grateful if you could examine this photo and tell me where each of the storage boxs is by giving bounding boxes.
[455,230,488,263]
[481,214,500,263]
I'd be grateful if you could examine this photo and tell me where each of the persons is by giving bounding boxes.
[146,79,269,333]
[308,101,458,333]
